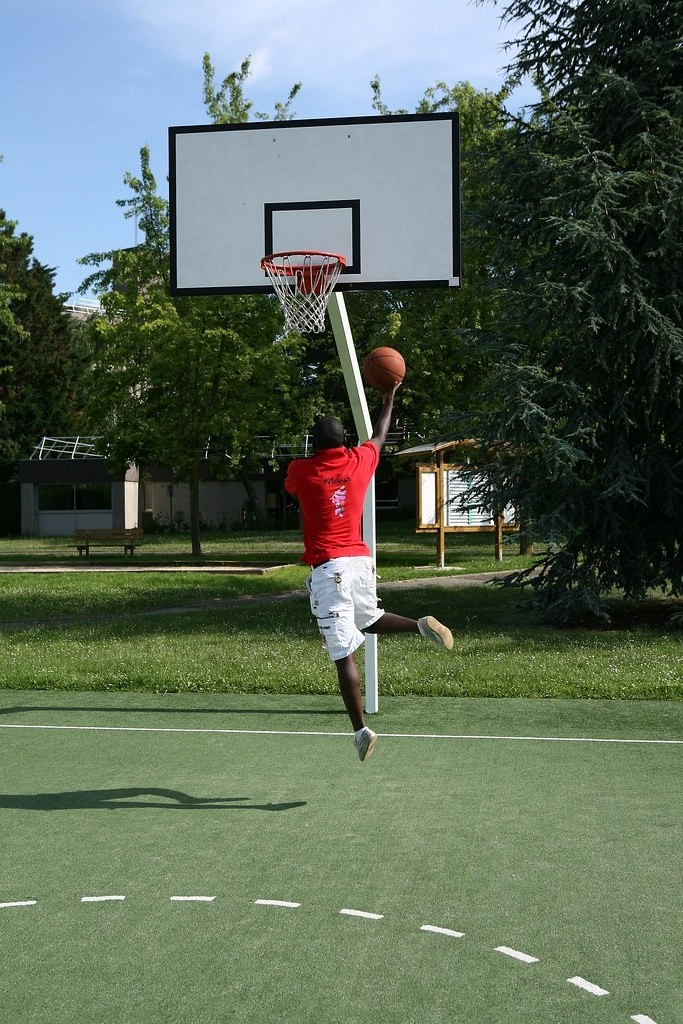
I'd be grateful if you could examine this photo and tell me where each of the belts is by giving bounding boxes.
[310,558,335,571]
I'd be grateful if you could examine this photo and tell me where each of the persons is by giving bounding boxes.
[285,381,454,762]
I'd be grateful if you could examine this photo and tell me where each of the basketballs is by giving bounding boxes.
[364,347,406,391]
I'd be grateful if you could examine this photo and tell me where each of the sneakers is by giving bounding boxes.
[419,616,454,650]
[353,728,377,761]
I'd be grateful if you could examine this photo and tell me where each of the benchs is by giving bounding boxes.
[68,527,141,558]
